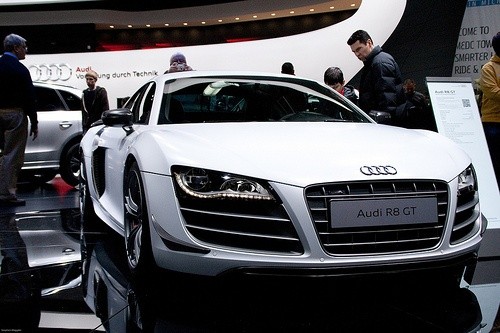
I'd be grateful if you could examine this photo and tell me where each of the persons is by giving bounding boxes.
[81,72,110,137]
[0,33,39,205]
[346,29,406,127]
[476,31,500,192]
[258,62,307,122]
[402,79,433,129]
[164,54,193,75]
[315,67,364,122]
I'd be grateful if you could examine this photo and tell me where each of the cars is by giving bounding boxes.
[20,80,86,188]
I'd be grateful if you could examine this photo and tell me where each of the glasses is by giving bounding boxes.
[20,45,28,50]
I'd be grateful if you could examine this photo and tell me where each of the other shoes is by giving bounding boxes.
[0,188,27,207]
[75,185,81,190]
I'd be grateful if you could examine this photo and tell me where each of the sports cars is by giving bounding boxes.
[78,70,489,290]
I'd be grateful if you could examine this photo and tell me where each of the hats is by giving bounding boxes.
[84,70,98,82]
